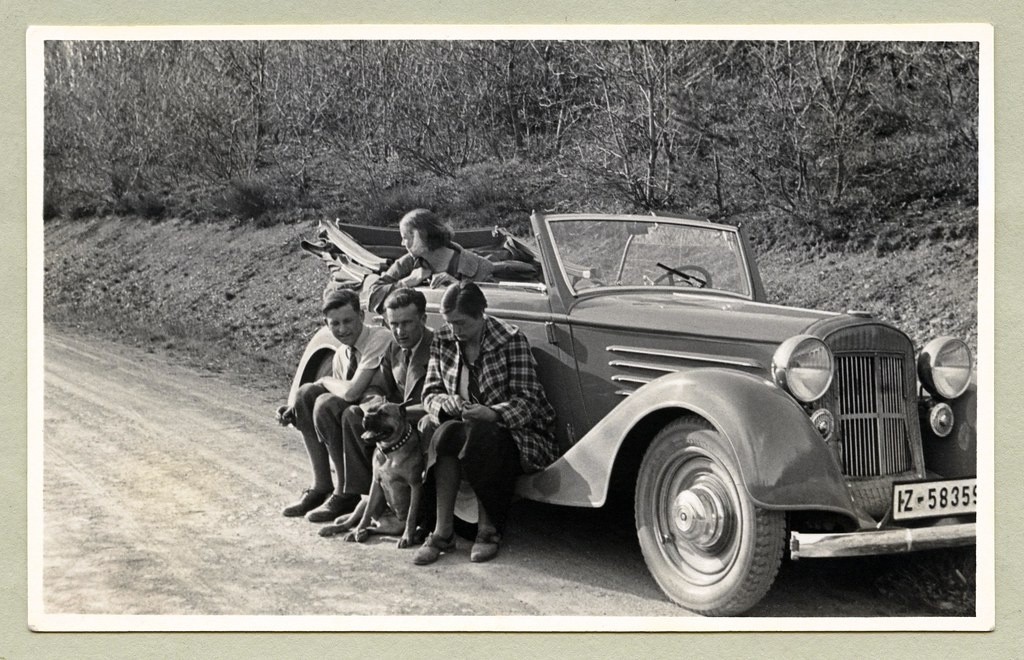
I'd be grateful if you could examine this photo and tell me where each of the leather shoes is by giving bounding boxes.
[284,488,335,516]
[306,494,362,521]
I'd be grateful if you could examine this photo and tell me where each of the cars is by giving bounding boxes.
[282,208,978,615]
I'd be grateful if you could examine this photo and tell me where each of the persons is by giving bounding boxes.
[342,286,436,503]
[363,208,495,315]
[274,290,402,523]
[412,279,560,565]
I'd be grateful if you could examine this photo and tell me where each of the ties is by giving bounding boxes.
[398,349,412,397]
[347,346,358,380]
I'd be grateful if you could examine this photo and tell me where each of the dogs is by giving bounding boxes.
[317,397,435,549]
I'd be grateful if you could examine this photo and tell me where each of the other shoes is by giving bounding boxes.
[470,531,501,561]
[412,523,428,544]
[412,532,457,564]
